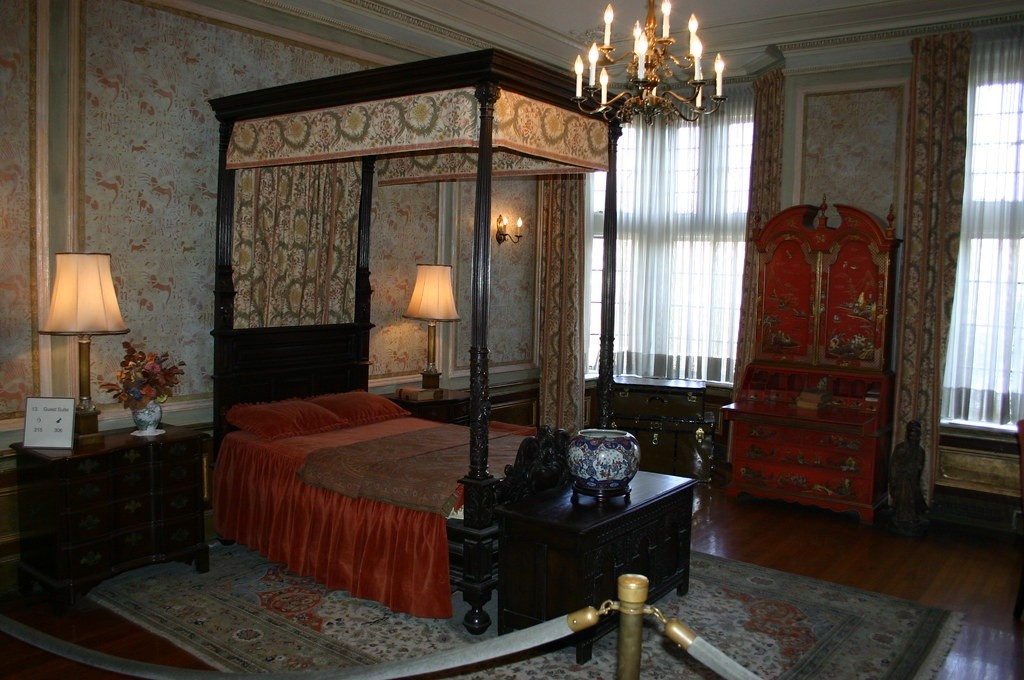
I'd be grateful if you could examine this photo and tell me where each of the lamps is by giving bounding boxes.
[570,0,728,127]
[402,263,461,389]
[496,214,523,245]
[38,251,131,435]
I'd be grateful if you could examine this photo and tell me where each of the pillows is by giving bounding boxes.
[226,397,351,442]
[304,388,410,428]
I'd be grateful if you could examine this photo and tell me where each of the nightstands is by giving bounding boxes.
[8,423,212,613]
[384,391,475,427]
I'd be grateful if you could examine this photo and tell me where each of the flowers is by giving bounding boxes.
[96,340,188,409]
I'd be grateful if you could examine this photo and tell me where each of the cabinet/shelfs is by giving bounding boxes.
[719,189,895,525]
[492,470,699,665]
[584,373,733,483]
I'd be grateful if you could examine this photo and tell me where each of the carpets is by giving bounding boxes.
[87,542,967,680]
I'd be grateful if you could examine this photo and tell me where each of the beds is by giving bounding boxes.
[205,48,698,665]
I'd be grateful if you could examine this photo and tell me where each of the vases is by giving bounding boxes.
[128,400,167,436]
